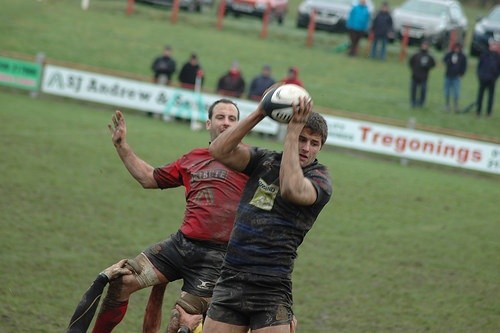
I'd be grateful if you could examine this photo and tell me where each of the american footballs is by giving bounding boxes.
[261,84,314,123]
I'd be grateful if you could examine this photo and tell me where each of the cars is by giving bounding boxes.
[388,0,469,52]
[297,0,376,34]
[223,0,289,25]
[471,4,500,57]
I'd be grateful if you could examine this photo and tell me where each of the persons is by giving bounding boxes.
[370,1,396,60]
[175,53,203,123]
[209,79,332,333]
[216,62,303,102]
[407,40,436,108]
[147,45,177,118]
[66,258,204,333]
[347,1,372,56]
[442,43,467,114]
[475,41,500,118]
[90,98,248,333]
